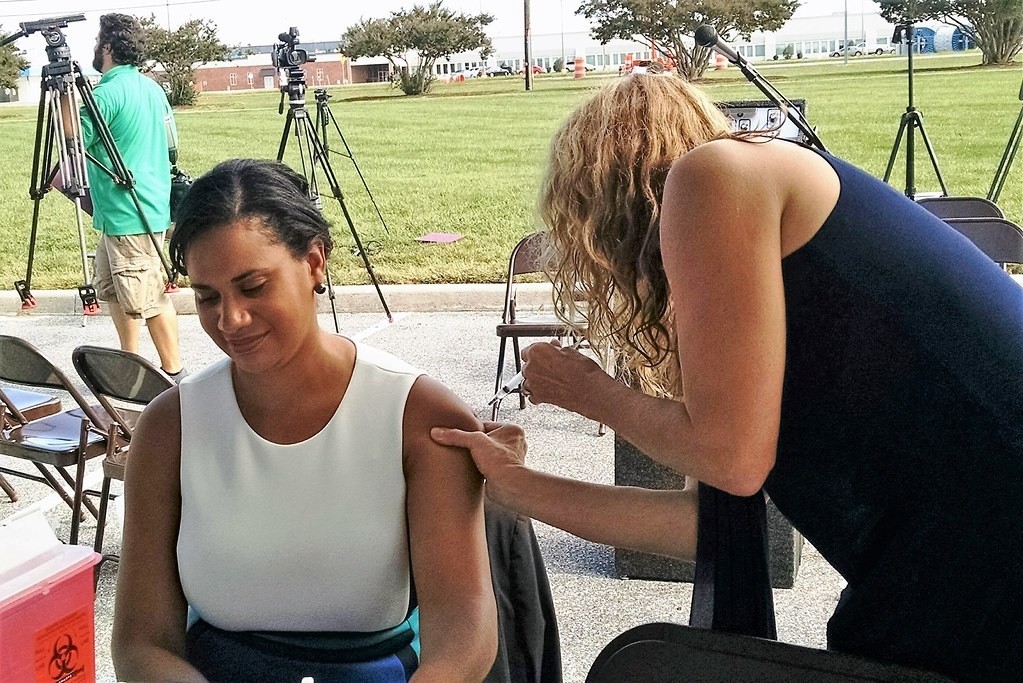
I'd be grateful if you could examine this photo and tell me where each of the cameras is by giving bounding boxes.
[271,26,316,68]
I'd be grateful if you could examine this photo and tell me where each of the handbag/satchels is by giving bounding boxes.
[170,170,195,222]
[159,366,189,384]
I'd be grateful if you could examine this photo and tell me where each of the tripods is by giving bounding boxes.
[0,14,180,316]
[274,68,394,335]
[882,19,948,205]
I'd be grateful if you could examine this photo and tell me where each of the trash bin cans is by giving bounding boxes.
[0,512,104,683]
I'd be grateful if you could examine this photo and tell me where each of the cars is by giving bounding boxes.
[517,65,545,77]
[477,65,510,79]
[565,62,595,73]
[449,69,478,80]
[856,43,888,56]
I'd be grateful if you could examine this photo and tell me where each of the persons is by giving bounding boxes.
[59,14,190,383]
[431,72,1023,683]
[112,159,498,683]
[619,65,625,76]
[5,85,17,102]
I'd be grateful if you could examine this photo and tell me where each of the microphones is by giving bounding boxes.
[696,26,757,74]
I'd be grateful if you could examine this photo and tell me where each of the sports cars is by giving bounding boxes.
[828,40,870,57]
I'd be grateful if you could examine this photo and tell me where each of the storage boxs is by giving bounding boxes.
[0,537,106,683]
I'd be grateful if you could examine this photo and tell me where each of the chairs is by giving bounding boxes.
[0,335,145,551]
[72,345,180,596]
[490,231,617,438]
[582,622,959,683]
[912,196,1022,290]
